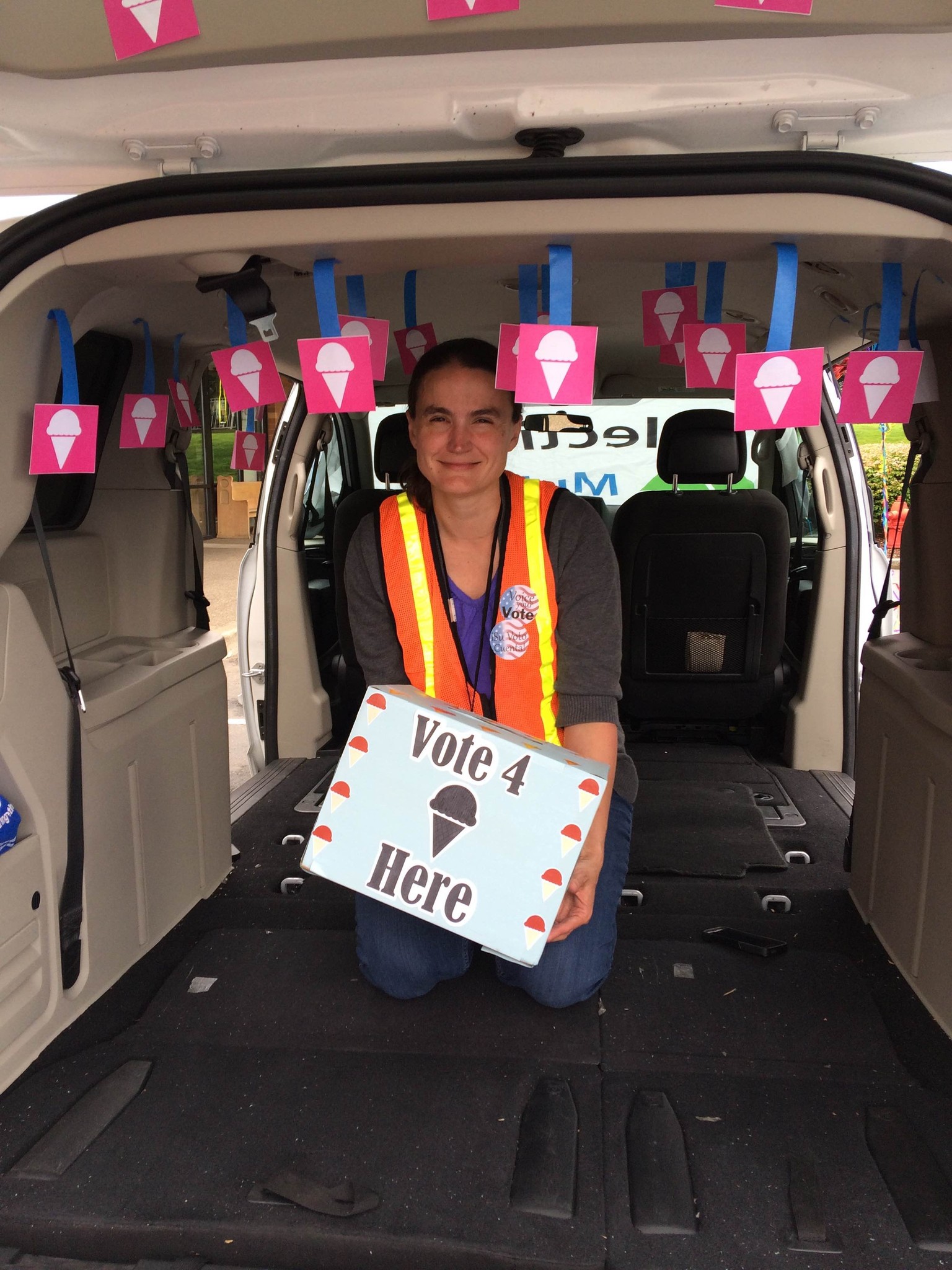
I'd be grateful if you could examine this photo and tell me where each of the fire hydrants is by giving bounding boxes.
[885,495,910,549]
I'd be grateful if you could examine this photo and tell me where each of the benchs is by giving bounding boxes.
[216,474,263,540]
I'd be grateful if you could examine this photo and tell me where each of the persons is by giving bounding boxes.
[345,337,639,1006]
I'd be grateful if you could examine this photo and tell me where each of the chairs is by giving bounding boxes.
[609,409,790,743]
[331,413,416,689]
